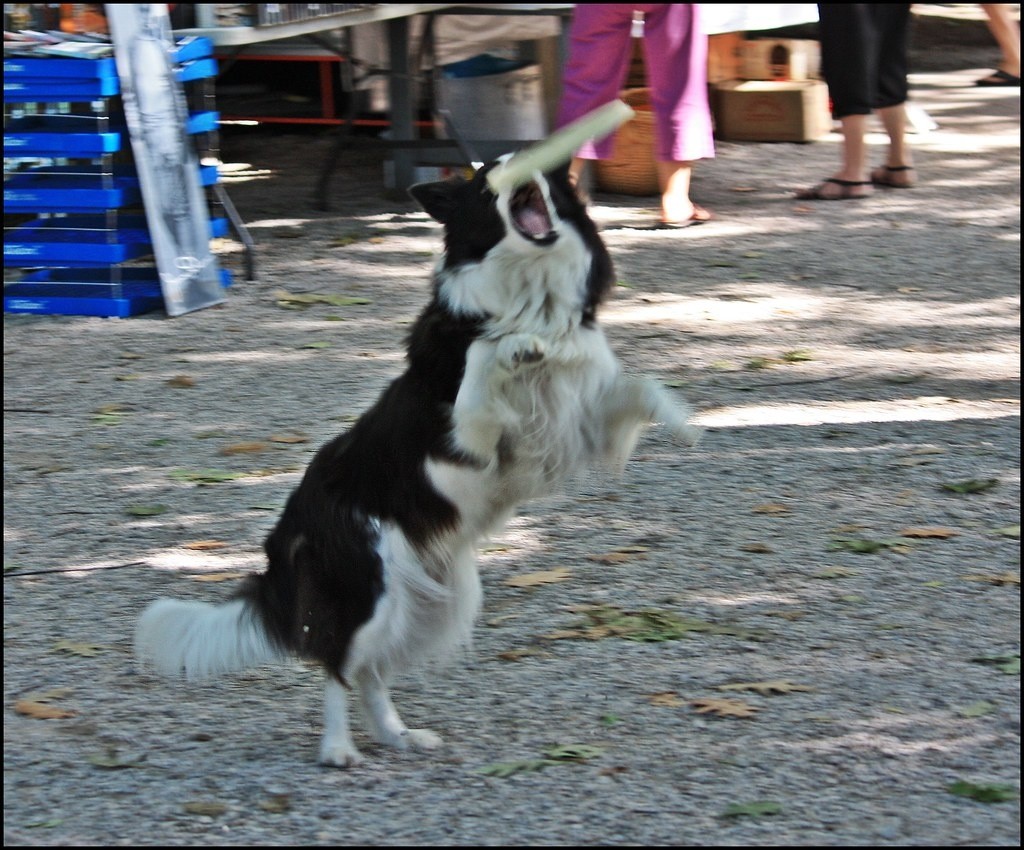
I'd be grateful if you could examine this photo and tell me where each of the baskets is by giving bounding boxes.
[590,87,716,196]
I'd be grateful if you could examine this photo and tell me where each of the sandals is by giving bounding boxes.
[872,165,920,188]
[795,178,875,199]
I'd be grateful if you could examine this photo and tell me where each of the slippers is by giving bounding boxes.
[975,71,1021,86]
[659,203,714,230]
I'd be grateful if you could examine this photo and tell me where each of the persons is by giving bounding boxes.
[973,3,1021,87]
[793,3,916,201]
[553,4,715,229]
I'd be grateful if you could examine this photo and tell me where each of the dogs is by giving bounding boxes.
[130,145,707,768]
